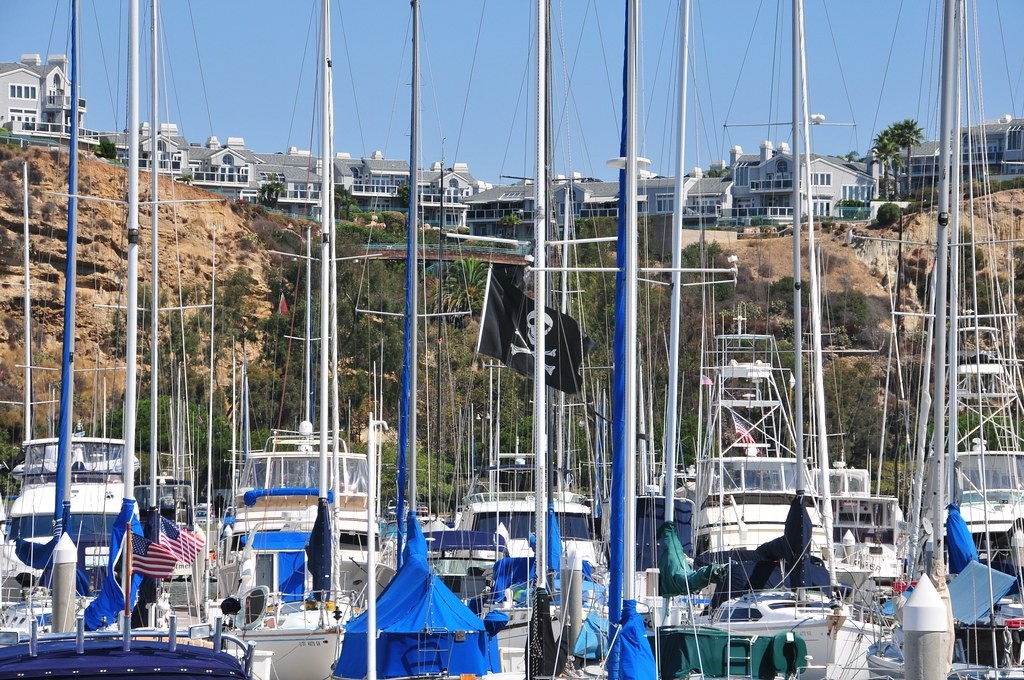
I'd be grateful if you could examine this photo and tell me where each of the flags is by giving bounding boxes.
[732,413,761,454]
[158,515,205,566]
[789,372,795,387]
[701,375,714,386]
[129,533,178,580]
[477,267,590,394]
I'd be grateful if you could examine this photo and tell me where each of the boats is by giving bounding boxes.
[1,0,1024,680]
